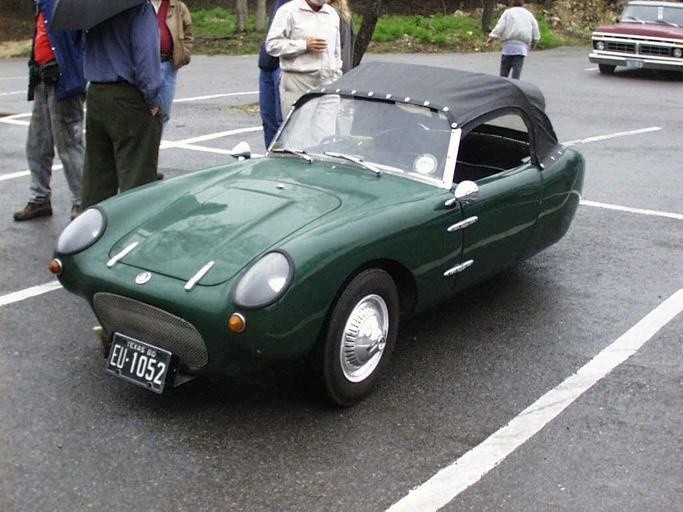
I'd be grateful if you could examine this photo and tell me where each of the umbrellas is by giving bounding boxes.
[44,0,149,37]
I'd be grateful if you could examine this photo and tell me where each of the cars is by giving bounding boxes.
[587,0,683,82]
[48,59,581,414]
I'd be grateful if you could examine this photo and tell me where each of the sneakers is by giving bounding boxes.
[71,204,80,219]
[14,201,52,220]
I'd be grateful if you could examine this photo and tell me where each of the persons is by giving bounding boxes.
[78,1,165,212]
[264,0,344,152]
[146,1,195,181]
[256,0,293,151]
[483,0,541,80]
[11,1,87,222]
[325,0,356,141]
